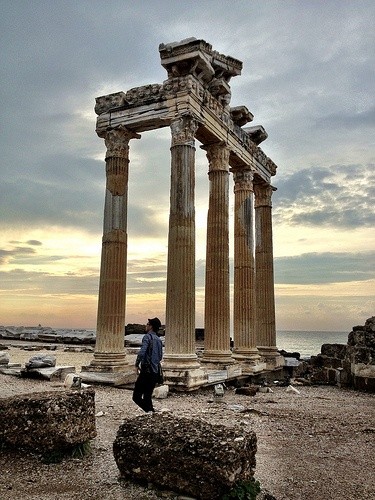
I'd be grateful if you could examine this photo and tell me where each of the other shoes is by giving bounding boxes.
[145,406,154,415]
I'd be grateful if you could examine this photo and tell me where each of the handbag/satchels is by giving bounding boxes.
[158,373,163,385]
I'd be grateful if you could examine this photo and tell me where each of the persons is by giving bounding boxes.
[130,317,163,412]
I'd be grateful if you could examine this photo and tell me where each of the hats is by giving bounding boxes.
[148,317,161,332]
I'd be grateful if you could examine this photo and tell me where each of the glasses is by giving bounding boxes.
[147,323,150,325]
